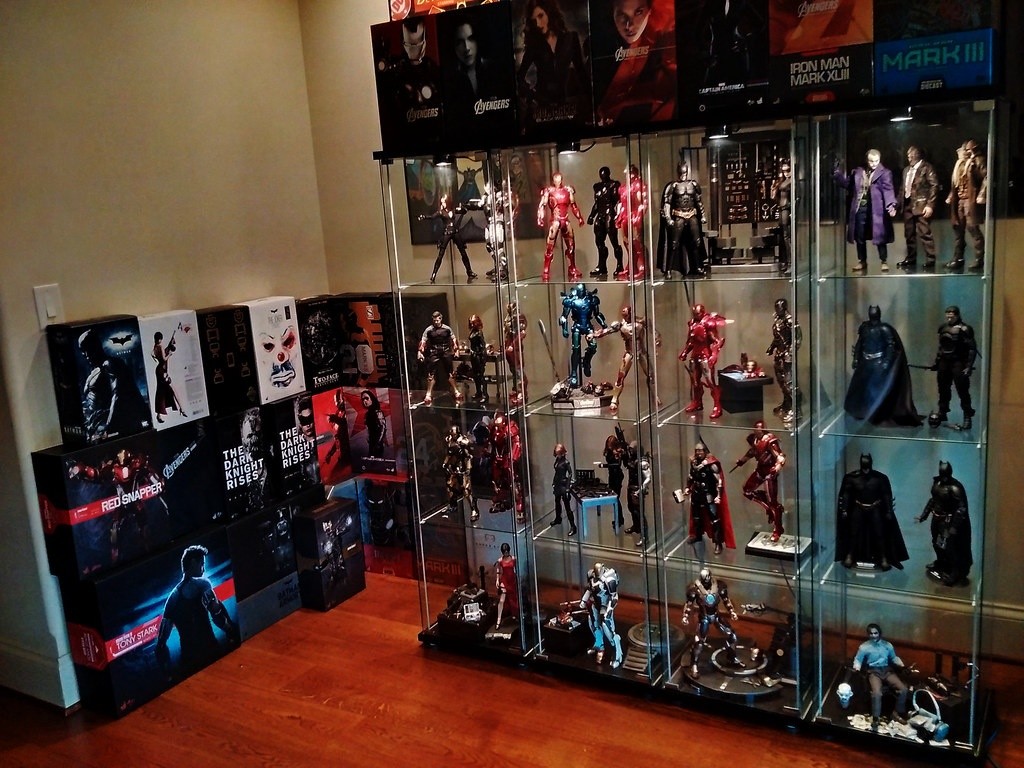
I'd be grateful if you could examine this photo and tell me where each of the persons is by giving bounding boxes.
[79,319,393,669]
[376,0,677,128]
[414,134,986,729]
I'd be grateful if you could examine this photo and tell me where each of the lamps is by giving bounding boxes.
[433,152,455,167]
[888,106,914,122]
[703,126,731,140]
[556,139,580,155]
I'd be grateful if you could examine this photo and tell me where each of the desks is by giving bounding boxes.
[570,478,621,540]
[452,351,513,399]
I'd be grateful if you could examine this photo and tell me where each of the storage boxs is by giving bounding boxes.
[292,496,367,612]
[138,309,210,432]
[196,304,262,417]
[463,495,531,598]
[327,292,454,391]
[44,312,152,447]
[403,395,534,499]
[344,386,410,484]
[370,0,901,149]
[873,29,1004,107]
[237,296,307,406]
[31,419,218,579]
[207,389,321,525]
[226,488,327,644]
[296,292,344,395]
[312,387,354,486]
[59,524,242,720]
[410,483,469,587]
[354,472,416,580]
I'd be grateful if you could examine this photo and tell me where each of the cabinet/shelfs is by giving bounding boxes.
[371,96,1012,768]
